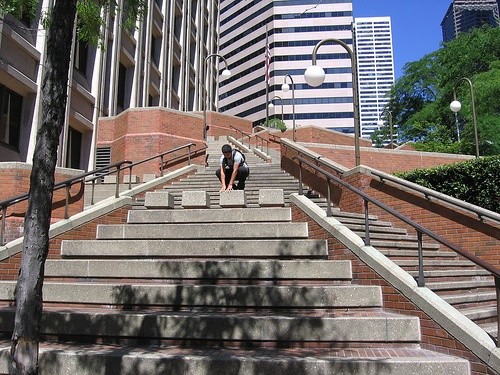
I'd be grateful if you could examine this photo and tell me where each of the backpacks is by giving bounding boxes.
[223,148,245,168]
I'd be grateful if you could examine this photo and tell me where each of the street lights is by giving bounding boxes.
[282,74,296,142]
[202,54,231,141]
[450,77,480,159]
[303,37,360,168]
[268,96,284,123]
[377,108,394,150]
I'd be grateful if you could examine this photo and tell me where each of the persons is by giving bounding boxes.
[215,144,250,193]
